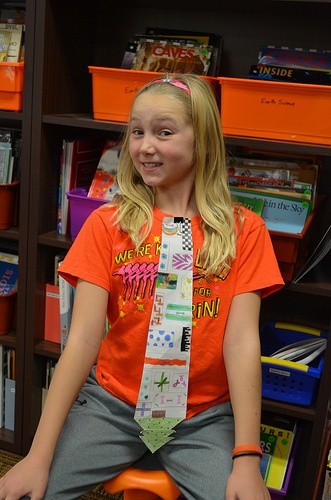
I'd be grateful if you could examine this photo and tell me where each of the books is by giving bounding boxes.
[6,348,15,380]
[121,25,331,87]
[260,424,294,491]
[268,336,327,366]
[292,224,331,284]
[56,135,320,236]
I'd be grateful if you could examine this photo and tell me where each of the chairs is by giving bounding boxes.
[102,467,180,500]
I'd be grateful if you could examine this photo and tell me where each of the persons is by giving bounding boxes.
[0,72,285,500]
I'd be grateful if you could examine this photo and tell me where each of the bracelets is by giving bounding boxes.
[232,445,263,459]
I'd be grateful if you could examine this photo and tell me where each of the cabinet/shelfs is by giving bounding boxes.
[0,0,331,500]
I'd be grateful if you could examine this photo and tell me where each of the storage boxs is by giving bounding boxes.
[258,323,327,406]
[219,77,331,148]
[89,66,217,134]
[43,283,64,346]
[266,211,317,264]
[0,61,23,111]
[253,415,301,492]
[66,189,111,239]
[1,286,18,336]
[0,180,19,229]
